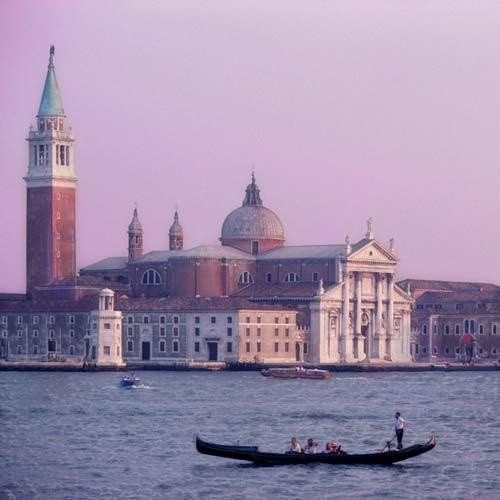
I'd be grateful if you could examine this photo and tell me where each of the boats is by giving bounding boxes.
[120,378,141,388]
[196,435,436,466]
[260,367,332,379]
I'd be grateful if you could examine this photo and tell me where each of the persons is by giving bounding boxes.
[288,437,301,452]
[322,442,332,453]
[328,443,342,454]
[392,412,407,450]
[121,372,140,381]
[303,437,320,454]
